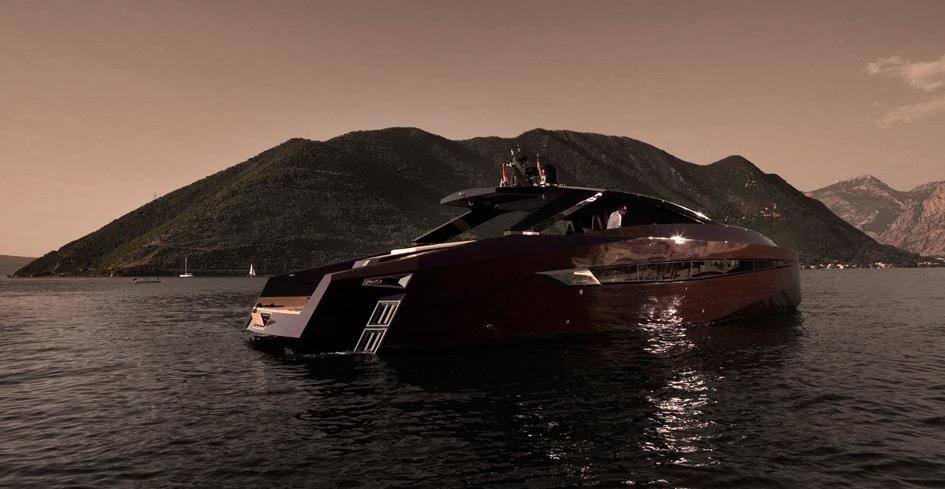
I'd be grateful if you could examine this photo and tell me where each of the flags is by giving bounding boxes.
[537,156,543,177]
[502,162,510,185]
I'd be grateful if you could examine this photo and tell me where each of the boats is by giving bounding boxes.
[242,162,803,360]
[134,278,161,284]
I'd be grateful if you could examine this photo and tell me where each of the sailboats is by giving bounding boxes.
[247,263,257,277]
[179,257,193,278]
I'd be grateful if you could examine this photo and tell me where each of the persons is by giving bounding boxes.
[606,204,628,229]
